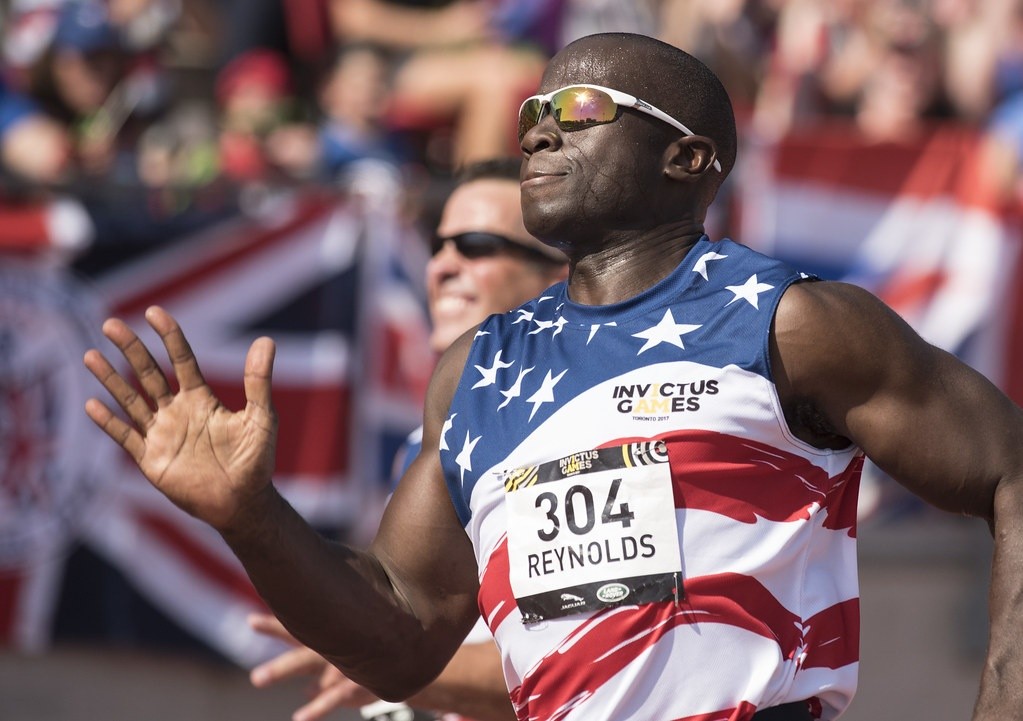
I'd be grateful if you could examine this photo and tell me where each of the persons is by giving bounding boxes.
[246,154,576,721]
[82,33,1023,721]
[0,1,1021,281]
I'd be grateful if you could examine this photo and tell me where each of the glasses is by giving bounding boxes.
[430,233,568,267]
[517,83,721,173]
